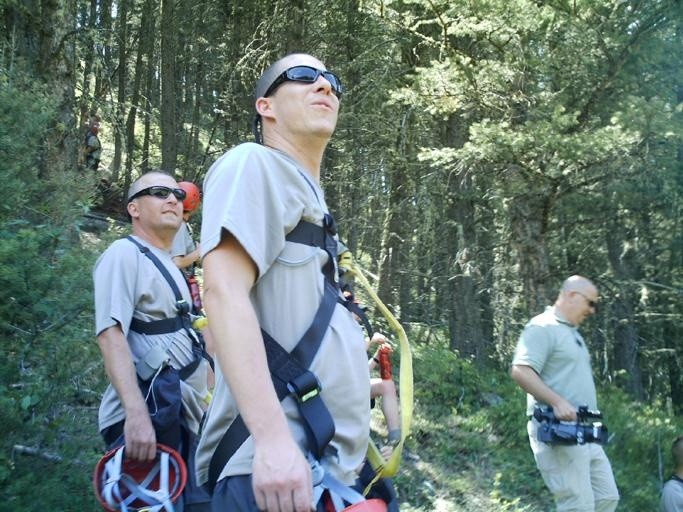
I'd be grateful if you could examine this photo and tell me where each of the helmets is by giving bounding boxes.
[94,443,188,512]
[178,181,200,211]
[339,499,389,512]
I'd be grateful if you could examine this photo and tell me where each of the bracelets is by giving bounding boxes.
[373,357,379,363]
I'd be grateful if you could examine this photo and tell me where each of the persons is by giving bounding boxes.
[659,437,683,512]
[92,171,207,512]
[351,300,419,461]
[194,53,388,512]
[170,181,215,390]
[510,274,620,512]
[86,122,102,172]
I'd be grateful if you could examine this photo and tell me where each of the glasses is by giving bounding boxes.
[576,291,598,308]
[262,64,344,98]
[127,185,186,204]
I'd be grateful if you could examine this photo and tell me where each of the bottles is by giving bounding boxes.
[188,275,201,310]
[379,348,391,380]
